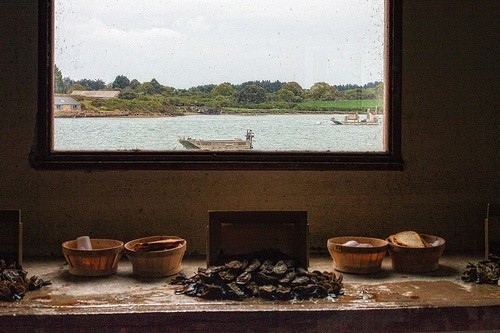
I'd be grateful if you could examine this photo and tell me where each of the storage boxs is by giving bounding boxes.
[206,209,309,270]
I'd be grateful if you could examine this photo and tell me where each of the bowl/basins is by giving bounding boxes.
[386,233,446,273]
[327,237,388,275]
[125,236,186,277]
[61,239,124,277]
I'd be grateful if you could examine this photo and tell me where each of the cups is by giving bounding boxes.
[77,236,92,251]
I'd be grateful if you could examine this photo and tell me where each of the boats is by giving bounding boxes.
[177,128,255,150]
[330,108,380,125]
